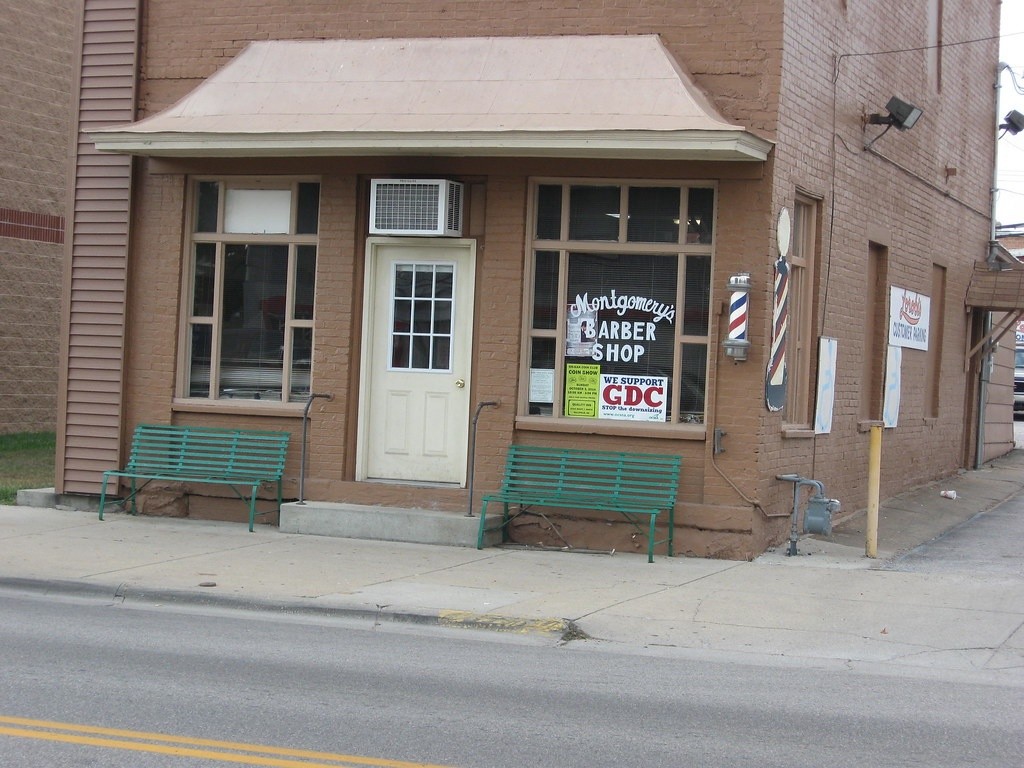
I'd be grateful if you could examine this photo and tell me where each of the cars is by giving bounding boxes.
[1014,346,1024,412]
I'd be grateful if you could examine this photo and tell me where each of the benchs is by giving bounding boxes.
[98,424,293,532]
[476,445,684,562]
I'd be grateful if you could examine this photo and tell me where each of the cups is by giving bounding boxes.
[940,491,956,500]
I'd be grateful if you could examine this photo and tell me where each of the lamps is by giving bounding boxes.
[999,110,1024,136]
[861,96,923,131]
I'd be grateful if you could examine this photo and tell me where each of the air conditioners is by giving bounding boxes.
[369,178,465,237]
